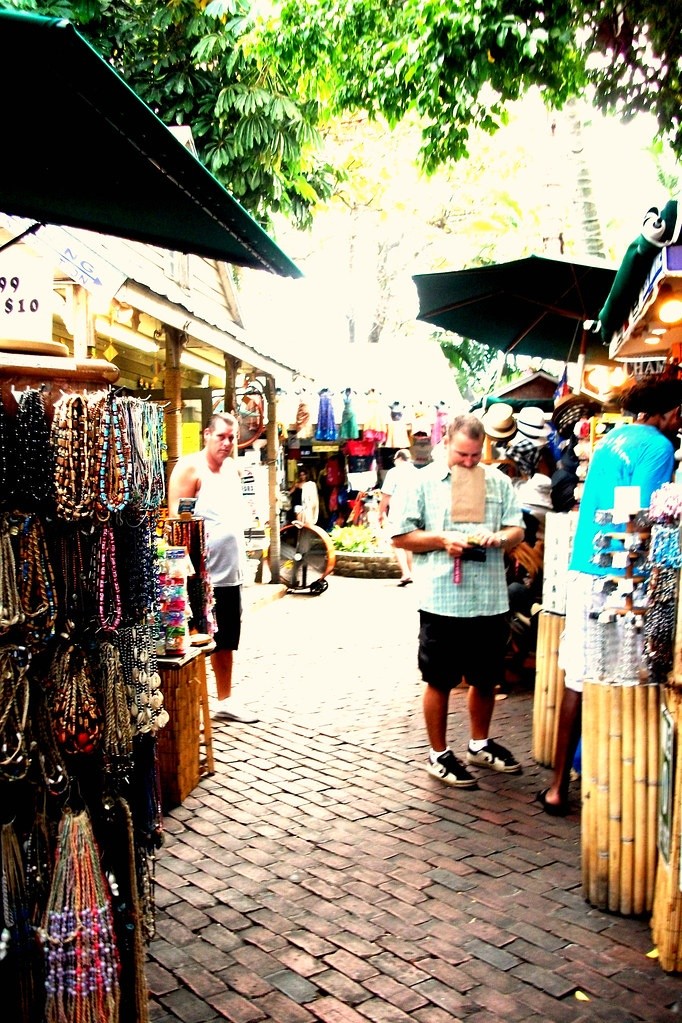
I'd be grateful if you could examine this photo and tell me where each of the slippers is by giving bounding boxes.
[536,786,572,817]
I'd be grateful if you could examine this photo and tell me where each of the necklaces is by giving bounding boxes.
[638,480,681,679]
[0,379,221,1023]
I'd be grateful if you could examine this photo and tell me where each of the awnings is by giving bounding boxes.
[1,4,303,290]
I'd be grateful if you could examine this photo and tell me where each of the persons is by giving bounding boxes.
[534,378,682,815]
[388,414,526,788]
[258,430,444,590]
[167,409,261,724]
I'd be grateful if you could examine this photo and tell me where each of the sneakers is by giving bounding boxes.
[466,738,523,773]
[427,750,478,787]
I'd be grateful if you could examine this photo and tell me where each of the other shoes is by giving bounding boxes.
[209,695,259,723]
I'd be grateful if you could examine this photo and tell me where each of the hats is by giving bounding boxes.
[511,406,553,437]
[549,392,589,424]
[480,402,517,442]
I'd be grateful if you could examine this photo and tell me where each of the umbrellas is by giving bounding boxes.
[407,254,621,386]
